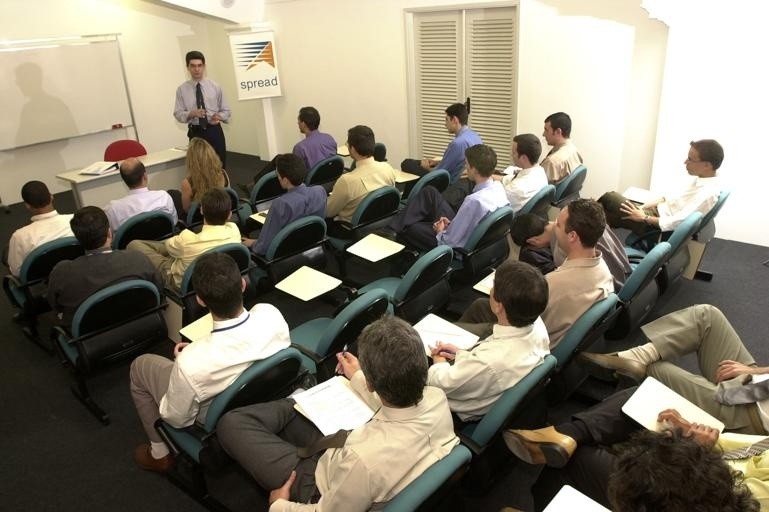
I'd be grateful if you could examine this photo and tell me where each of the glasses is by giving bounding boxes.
[688,156,701,162]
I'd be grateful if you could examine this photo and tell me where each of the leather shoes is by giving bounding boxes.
[503,426,577,468]
[134,443,178,474]
[578,352,646,386]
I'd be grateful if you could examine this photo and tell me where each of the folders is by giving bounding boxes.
[78,162,119,175]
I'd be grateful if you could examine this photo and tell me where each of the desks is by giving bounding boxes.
[55,146,187,209]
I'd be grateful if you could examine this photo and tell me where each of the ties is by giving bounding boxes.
[722,436,769,460]
[296,405,384,458]
[713,378,769,406]
[196,83,208,130]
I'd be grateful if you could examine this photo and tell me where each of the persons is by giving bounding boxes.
[101,157,178,240]
[366,144,511,262]
[573,304,769,435]
[501,385,769,511]
[237,107,337,199]
[596,139,724,243]
[7,181,76,278]
[241,154,328,255]
[216,316,461,511]
[453,198,615,350]
[326,125,396,240]
[401,103,483,199]
[539,113,583,187]
[441,134,549,220]
[129,252,291,473]
[425,259,551,422]
[127,188,243,296]
[512,214,633,294]
[167,137,230,224]
[173,51,231,169]
[46,206,164,331]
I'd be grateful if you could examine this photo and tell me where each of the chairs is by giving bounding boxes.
[49,280,170,422]
[164,243,256,345]
[625,191,731,282]
[337,143,387,173]
[177,188,244,228]
[304,156,344,186]
[454,355,557,456]
[345,245,453,322]
[551,165,587,209]
[515,185,556,221]
[239,170,287,214]
[624,212,703,292]
[112,211,180,250]
[551,292,618,366]
[250,216,329,291]
[287,288,388,378]
[607,242,671,341]
[452,206,513,282]
[330,186,400,276]
[104,139,147,162]
[154,348,310,512]
[379,445,472,511]
[400,169,450,206]
[2,236,85,356]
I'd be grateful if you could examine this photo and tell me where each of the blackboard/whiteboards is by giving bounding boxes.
[0,39,135,152]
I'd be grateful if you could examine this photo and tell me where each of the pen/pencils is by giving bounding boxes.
[335,345,348,373]
[440,349,456,355]
[201,105,203,118]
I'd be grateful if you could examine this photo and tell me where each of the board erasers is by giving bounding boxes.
[112,124,122,129]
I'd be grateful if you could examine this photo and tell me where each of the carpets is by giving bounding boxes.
[0,151,769,512]
